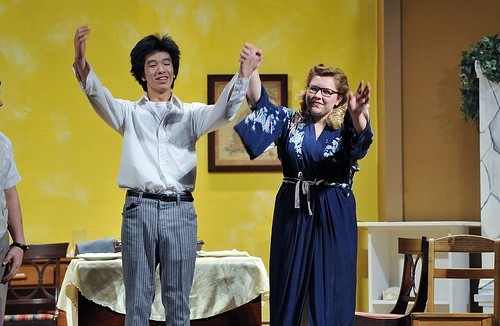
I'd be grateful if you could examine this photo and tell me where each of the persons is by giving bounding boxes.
[72,25,260,326]
[0,82,28,326]
[233,43,374,326]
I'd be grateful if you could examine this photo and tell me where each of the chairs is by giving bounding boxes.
[355,235,500,326]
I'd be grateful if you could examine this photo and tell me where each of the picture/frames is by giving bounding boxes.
[207,73,288,172]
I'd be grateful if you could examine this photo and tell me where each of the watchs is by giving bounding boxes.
[10,242,28,253]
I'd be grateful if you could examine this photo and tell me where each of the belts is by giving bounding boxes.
[126,190,194,202]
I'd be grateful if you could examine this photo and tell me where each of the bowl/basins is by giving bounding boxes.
[196,240,204,251]
[372,299,415,315]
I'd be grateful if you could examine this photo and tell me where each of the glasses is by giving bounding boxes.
[308,84,342,98]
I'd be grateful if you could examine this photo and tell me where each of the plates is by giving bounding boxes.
[75,252,123,261]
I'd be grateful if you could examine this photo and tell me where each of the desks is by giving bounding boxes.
[56,250,270,326]
